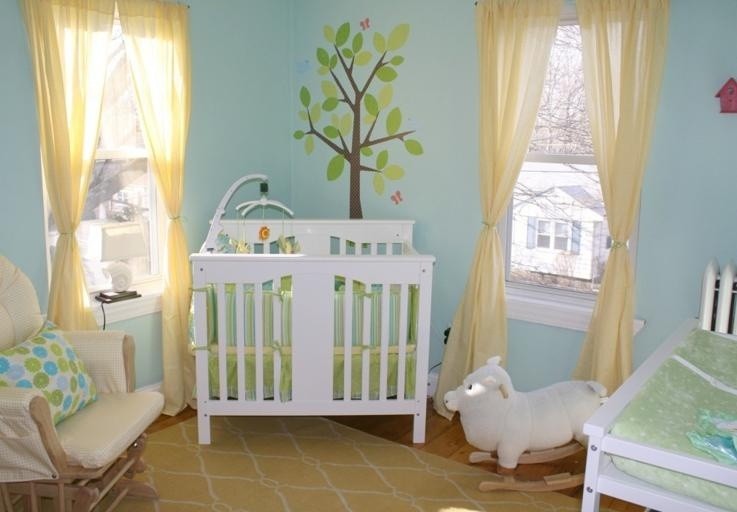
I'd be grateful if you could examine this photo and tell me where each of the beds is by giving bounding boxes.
[189,217,438,445]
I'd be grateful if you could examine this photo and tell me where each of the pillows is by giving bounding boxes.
[0,319,98,429]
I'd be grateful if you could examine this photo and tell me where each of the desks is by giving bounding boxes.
[579,329,736,512]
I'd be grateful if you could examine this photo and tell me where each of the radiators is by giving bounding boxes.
[697,256,737,335]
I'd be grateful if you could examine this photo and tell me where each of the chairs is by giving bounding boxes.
[0,255,166,512]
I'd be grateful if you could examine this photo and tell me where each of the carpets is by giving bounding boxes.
[103,416,616,512]
